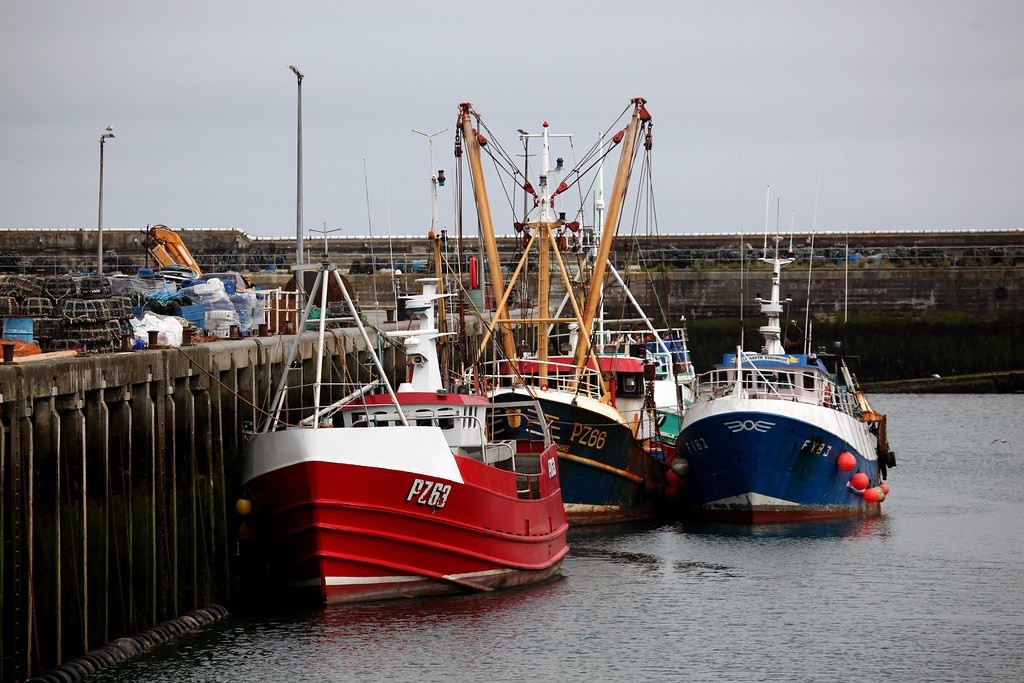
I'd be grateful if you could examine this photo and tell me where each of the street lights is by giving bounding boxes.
[96,124,117,274]
[288,64,306,330]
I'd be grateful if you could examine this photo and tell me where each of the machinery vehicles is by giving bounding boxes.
[143,224,202,281]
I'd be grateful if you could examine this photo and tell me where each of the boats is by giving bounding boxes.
[236,226,574,606]
[670,183,898,524]
[435,99,697,528]
